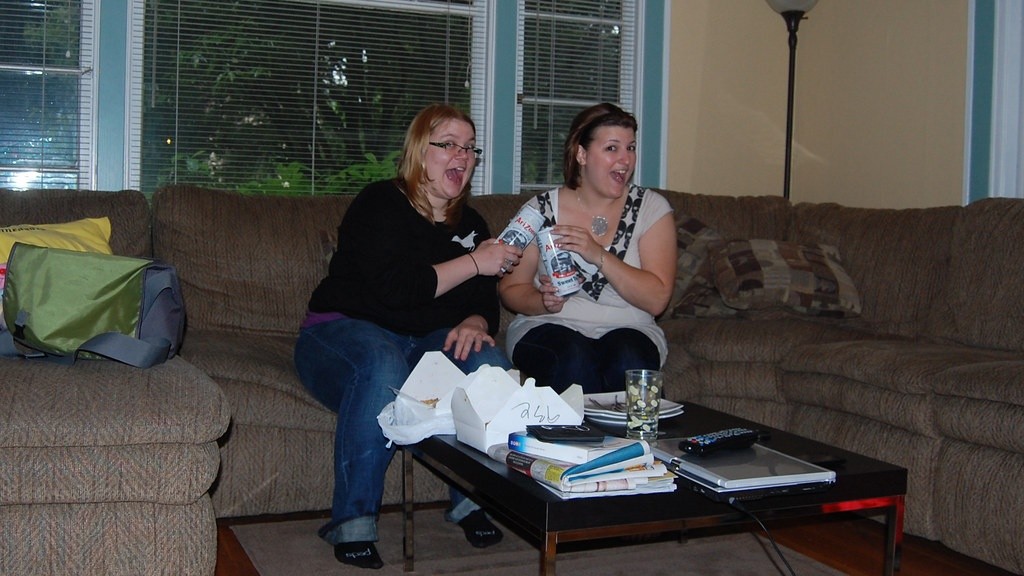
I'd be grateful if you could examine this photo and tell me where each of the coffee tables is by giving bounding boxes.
[401,408,908,576]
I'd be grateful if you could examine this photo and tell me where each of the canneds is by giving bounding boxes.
[493,204,546,272]
[536,227,580,297]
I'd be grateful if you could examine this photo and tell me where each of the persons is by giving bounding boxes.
[495,102,678,392]
[292,103,523,568]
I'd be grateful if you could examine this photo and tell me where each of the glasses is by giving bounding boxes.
[428,141,483,162]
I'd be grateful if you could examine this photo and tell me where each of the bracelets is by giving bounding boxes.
[599,245,603,269]
[467,253,480,275]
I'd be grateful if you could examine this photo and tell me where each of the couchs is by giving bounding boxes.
[652,189,1023,575]
[0,185,231,576]
[156,185,700,520]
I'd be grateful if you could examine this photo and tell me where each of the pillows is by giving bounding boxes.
[0,218,113,266]
[657,211,862,321]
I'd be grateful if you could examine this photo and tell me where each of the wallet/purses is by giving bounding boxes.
[526,422,606,443]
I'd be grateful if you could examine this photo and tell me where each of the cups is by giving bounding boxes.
[625,369,664,441]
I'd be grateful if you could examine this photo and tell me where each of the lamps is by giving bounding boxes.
[766,0,818,199]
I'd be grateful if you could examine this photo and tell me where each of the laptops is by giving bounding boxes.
[649,437,838,501]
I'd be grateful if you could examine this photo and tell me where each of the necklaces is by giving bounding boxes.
[575,189,608,236]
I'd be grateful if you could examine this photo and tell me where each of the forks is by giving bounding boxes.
[612,402,623,412]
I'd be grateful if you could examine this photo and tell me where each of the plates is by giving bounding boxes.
[583,391,683,425]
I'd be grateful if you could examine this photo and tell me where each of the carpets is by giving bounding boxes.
[230,507,845,575]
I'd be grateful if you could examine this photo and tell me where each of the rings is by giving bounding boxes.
[504,261,509,270]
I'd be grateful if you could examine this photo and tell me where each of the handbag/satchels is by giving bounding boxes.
[4,242,187,368]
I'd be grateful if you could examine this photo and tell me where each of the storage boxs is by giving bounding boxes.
[394,349,588,454]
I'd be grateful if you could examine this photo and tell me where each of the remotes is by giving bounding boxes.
[682,428,759,456]
